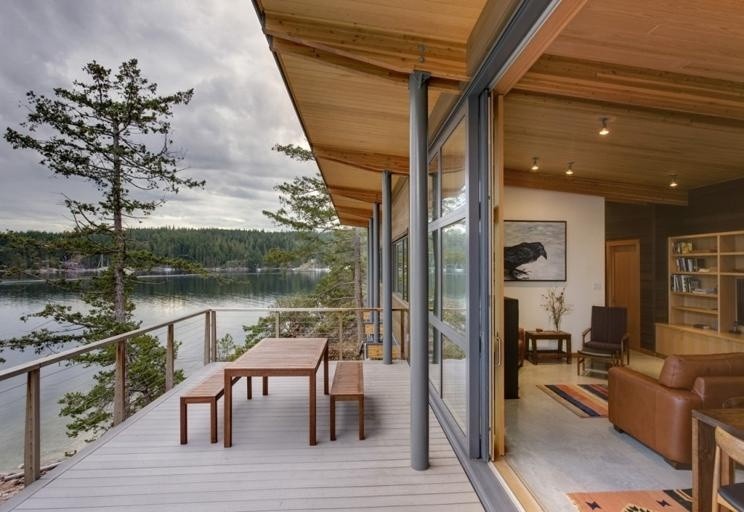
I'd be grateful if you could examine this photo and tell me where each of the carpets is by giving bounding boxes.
[535,382,608,419]
[563,488,692,511]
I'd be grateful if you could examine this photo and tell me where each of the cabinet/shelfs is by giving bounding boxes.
[667,230,743,340]
[654,321,744,360]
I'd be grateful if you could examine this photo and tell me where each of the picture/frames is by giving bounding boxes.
[502,219,567,283]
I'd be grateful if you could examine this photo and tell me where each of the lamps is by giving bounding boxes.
[598,118,610,136]
[529,157,540,171]
[668,175,679,188]
[565,161,577,176]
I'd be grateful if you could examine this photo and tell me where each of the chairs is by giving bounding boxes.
[711,426,744,512]
[576,306,632,376]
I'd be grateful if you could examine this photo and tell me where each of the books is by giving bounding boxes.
[670,242,716,295]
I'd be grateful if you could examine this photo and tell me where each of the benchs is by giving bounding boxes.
[176,368,251,448]
[327,359,369,442]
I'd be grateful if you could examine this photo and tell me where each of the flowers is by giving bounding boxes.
[539,286,569,332]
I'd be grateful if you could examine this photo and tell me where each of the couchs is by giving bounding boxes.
[605,352,743,473]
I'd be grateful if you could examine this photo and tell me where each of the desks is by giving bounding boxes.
[688,405,743,512]
[221,334,331,448]
[524,330,572,366]
